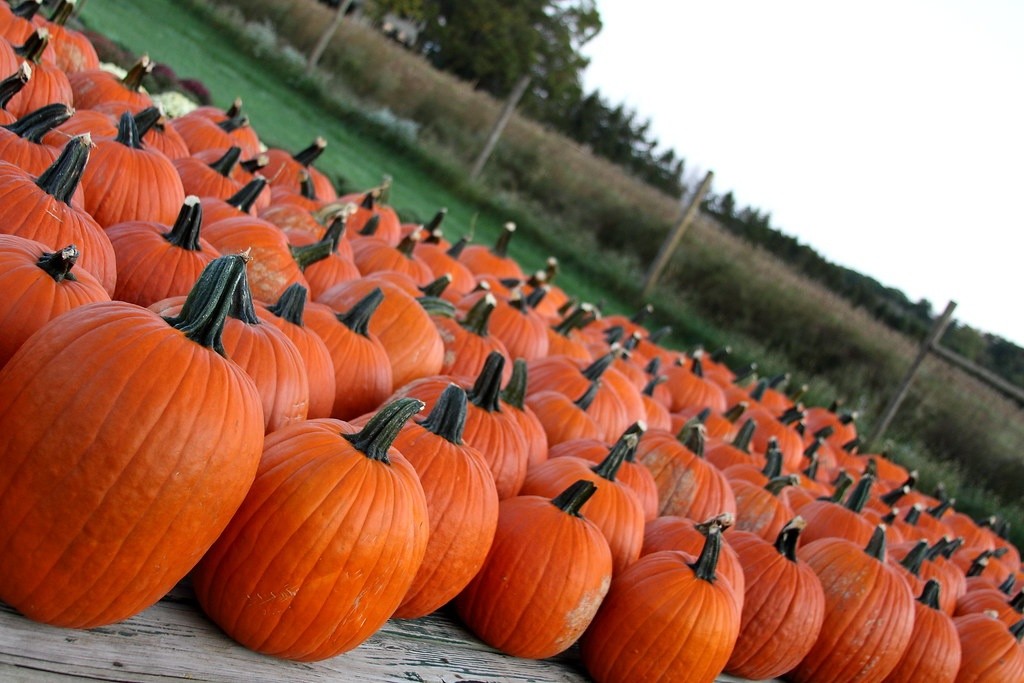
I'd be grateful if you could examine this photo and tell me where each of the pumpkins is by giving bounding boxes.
[2,0,1024,683]
